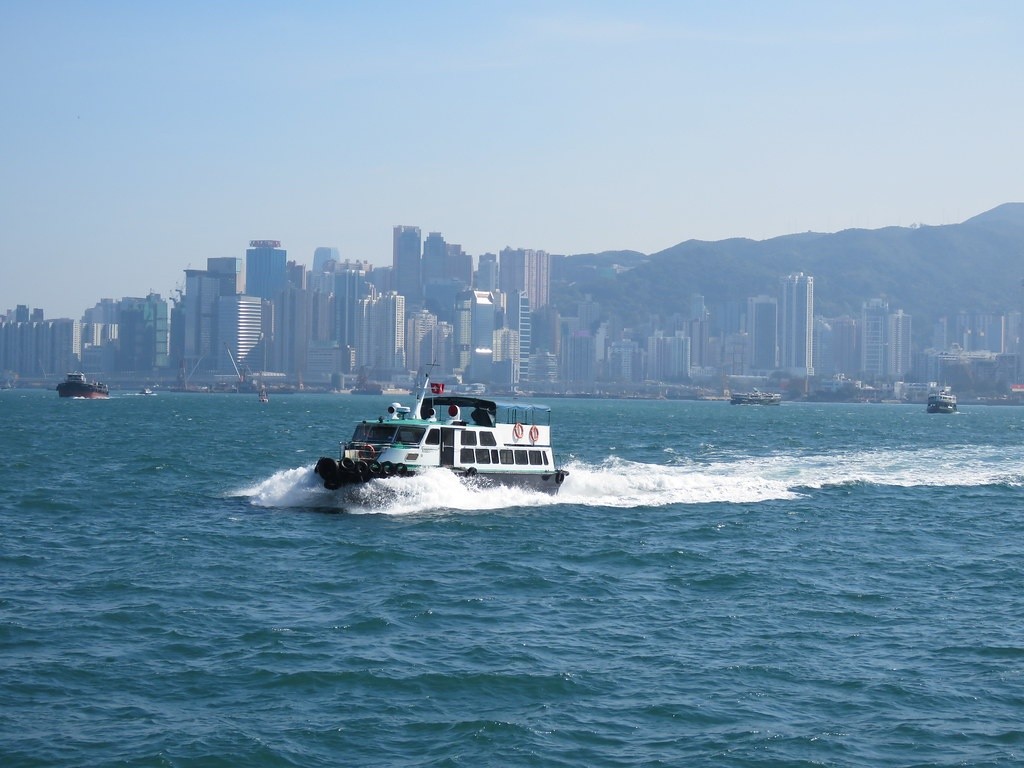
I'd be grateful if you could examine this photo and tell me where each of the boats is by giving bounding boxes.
[255,388,270,403]
[929,386,956,415]
[129,385,157,398]
[728,388,781,406]
[311,360,569,503]
[51,370,111,401]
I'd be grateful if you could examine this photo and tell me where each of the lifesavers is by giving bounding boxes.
[514,423,524,438]
[530,425,539,441]
[359,445,375,458]
